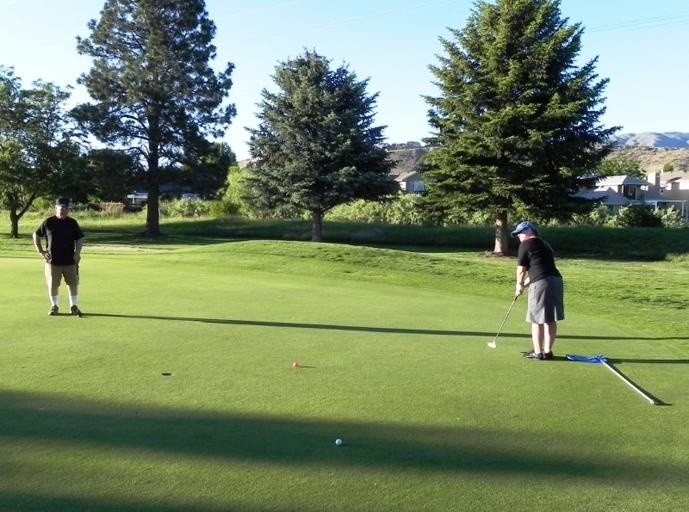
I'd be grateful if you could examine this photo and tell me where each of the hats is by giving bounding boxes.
[512,221,535,237]
[56,197,68,206]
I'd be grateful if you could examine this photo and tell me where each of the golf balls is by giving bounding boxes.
[334,438,343,445]
[293,361,300,368]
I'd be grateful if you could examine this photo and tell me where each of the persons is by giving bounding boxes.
[32,197,84,315]
[511,222,565,361]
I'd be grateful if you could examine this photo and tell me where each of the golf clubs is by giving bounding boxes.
[75,260,83,318]
[487,291,519,348]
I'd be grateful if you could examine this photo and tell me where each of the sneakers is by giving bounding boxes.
[49,306,57,315]
[71,306,80,314]
[527,352,553,360]
